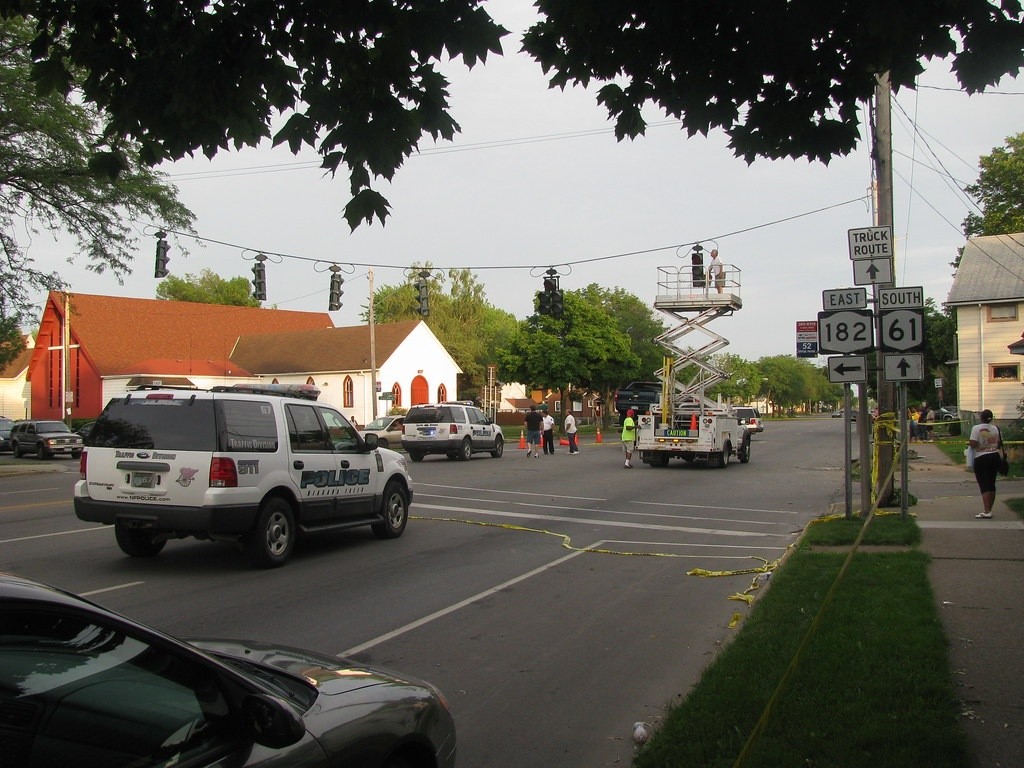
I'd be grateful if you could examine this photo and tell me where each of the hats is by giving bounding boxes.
[981,409,993,422]
[627,410,634,418]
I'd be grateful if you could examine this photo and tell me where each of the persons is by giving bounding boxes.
[907,404,935,443]
[562,408,580,455]
[393,419,403,431]
[349,416,362,431]
[541,410,555,454]
[524,405,544,458]
[969,409,1003,520]
[709,249,726,294]
[872,408,878,420]
[621,409,641,469]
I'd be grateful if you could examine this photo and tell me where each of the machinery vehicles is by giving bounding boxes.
[634,265,752,468]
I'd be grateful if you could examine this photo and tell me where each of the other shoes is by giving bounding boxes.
[567,452,575,454]
[573,451,579,454]
[624,465,632,468]
[550,452,554,454]
[527,449,532,457]
[544,452,548,454]
[534,453,539,458]
[974,512,991,518]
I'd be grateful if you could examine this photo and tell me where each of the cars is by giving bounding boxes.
[851,410,859,421]
[935,406,957,422]
[355,415,406,449]
[71,422,98,457]
[0,572,459,768]
[831,411,842,418]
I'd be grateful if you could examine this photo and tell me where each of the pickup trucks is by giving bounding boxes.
[614,381,666,423]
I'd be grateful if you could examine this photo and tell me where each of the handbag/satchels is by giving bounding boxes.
[998,457,1010,476]
[964,444,975,473]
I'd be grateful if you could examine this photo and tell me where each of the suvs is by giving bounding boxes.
[401,400,505,460]
[74,383,414,568]
[0,416,14,450]
[731,405,764,432]
[10,421,83,458]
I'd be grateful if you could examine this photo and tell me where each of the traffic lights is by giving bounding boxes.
[155,240,171,279]
[252,262,267,299]
[538,276,563,315]
[414,281,430,317]
[329,274,344,310]
[692,253,705,287]
